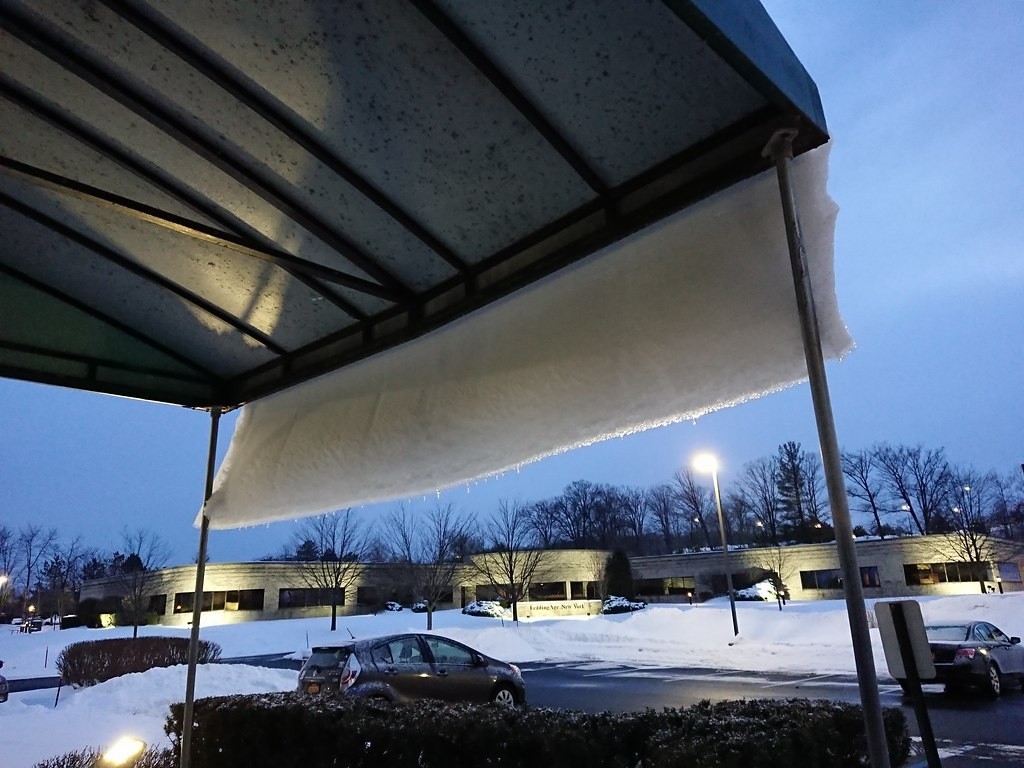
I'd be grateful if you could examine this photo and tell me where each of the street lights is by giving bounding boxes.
[691,452,739,635]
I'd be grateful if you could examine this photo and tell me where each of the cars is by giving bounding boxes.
[295,632,527,708]
[896,619,1024,704]
